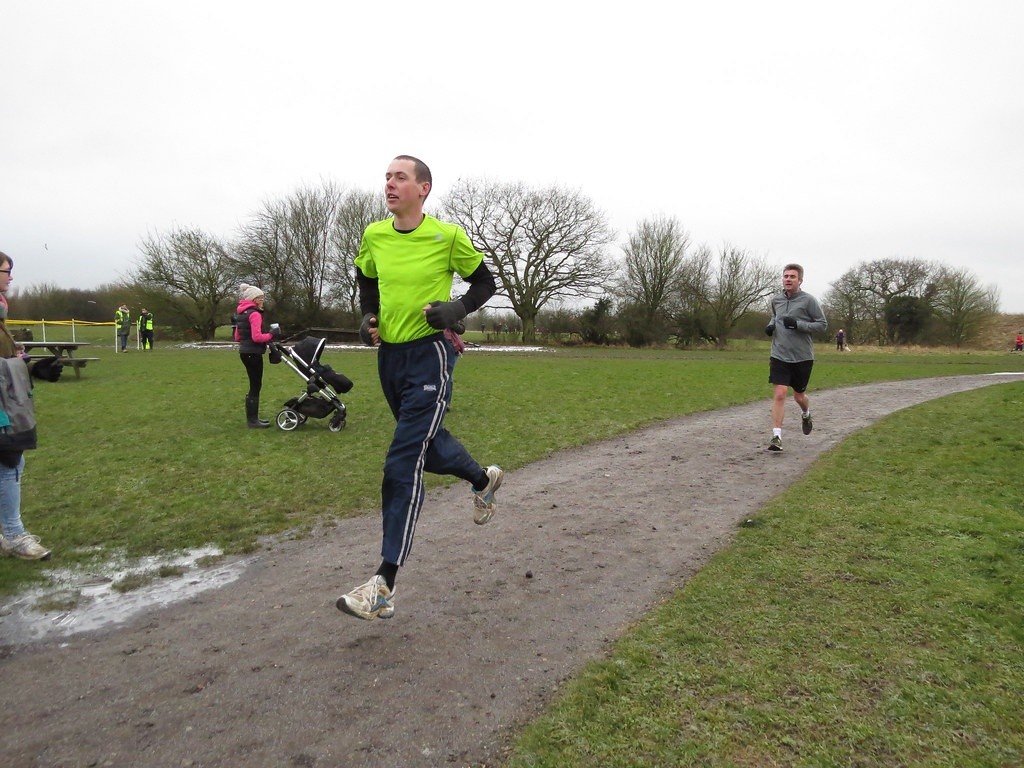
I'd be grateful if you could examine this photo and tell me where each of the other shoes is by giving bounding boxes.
[1,532,51,561]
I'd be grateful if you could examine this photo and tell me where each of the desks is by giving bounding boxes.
[17,342,90,357]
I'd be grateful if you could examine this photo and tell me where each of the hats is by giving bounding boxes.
[240,283,264,301]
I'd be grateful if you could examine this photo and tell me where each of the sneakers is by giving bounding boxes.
[801,413,813,434]
[337,574,397,621]
[471,463,504,525]
[767,436,783,451]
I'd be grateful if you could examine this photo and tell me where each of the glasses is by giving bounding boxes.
[0,270,11,277]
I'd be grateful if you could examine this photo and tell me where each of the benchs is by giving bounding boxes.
[26,354,100,379]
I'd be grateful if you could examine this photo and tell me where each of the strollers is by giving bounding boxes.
[266,336,353,432]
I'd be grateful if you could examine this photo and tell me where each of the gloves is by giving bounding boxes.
[765,325,775,336]
[269,327,281,337]
[783,317,797,329]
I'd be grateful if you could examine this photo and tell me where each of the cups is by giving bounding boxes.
[270,323,279,329]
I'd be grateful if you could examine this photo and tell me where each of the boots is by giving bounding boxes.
[246,394,270,428]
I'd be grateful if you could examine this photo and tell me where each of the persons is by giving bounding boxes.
[836,329,851,351]
[481,323,546,336]
[1011,332,1024,355]
[764,264,828,451]
[114,303,132,352]
[137,307,155,352]
[0,252,64,561]
[230,283,279,428]
[445,320,466,410]
[337,155,503,620]
